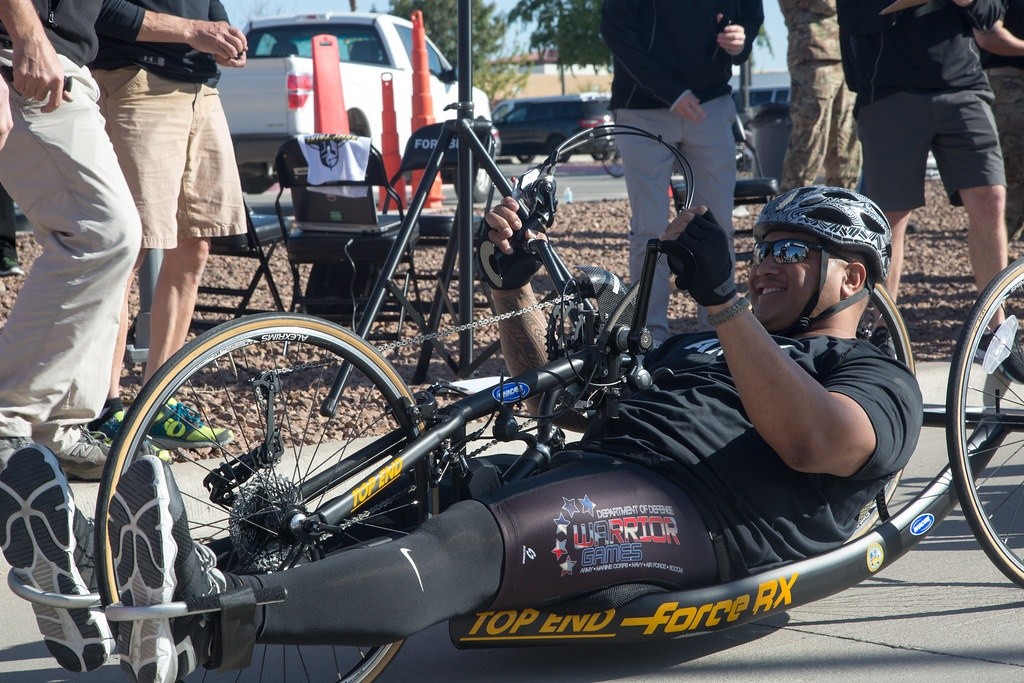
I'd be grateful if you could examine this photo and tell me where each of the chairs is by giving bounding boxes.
[349,41,380,62]
[270,41,299,57]
[381,116,498,320]
[272,133,425,359]
[126,196,300,343]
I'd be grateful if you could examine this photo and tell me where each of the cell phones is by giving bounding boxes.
[704,15,732,60]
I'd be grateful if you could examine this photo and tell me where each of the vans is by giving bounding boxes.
[731,86,791,132]
[491,92,612,122]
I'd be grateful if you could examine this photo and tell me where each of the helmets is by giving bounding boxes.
[751,186,893,283]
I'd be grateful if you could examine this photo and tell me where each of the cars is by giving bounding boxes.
[492,98,616,163]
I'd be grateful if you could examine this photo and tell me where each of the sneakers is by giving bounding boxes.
[107,455,227,683]
[976,324,1024,386]
[1,424,112,481]
[88,403,178,463]
[0,443,117,674]
[148,398,234,448]
[868,326,896,359]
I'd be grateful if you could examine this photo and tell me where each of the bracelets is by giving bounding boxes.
[707,297,749,327]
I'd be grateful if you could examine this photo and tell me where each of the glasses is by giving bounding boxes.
[753,239,822,265]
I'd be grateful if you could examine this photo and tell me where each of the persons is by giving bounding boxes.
[778,0,862,193]
[0,0,248,483]
[597,0,765,351]
[835,0,1024,384]
[0,186,924,683]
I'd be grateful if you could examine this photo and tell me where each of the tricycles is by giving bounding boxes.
[7,124,1024,683]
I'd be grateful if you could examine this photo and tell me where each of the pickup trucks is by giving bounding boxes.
[215,11,502,203]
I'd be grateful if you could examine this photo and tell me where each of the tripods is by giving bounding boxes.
[320,0,595,418]
